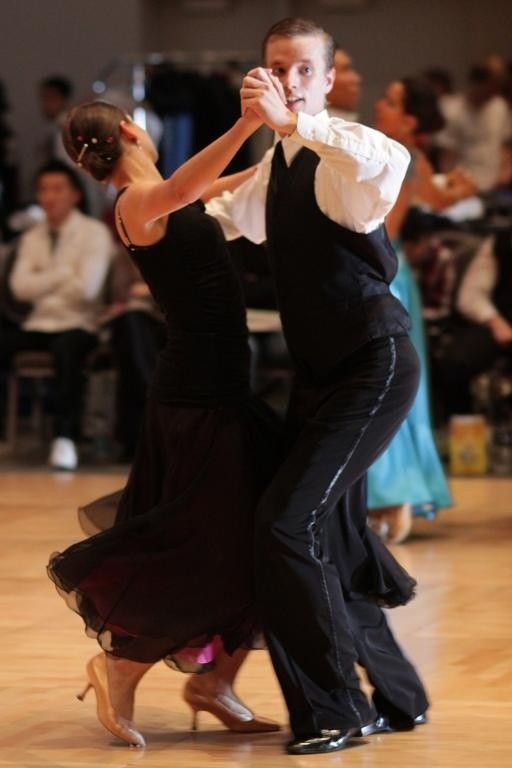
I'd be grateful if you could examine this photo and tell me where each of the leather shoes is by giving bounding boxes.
[375,710,424,731]
[284,717,374,756]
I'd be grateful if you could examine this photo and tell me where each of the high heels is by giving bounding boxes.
[368,503,432,543]
[77,651,146,747]
[182,681,281,734]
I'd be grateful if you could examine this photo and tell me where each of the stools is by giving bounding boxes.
[5,352,54,460]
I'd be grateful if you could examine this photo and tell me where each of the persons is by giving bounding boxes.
[2,59,250,470]
[187,11,433,757]
[41,64,291,749]
[328,42,510,465]
[340,69,483,549]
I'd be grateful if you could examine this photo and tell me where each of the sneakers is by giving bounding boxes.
[46,436,77,470]
